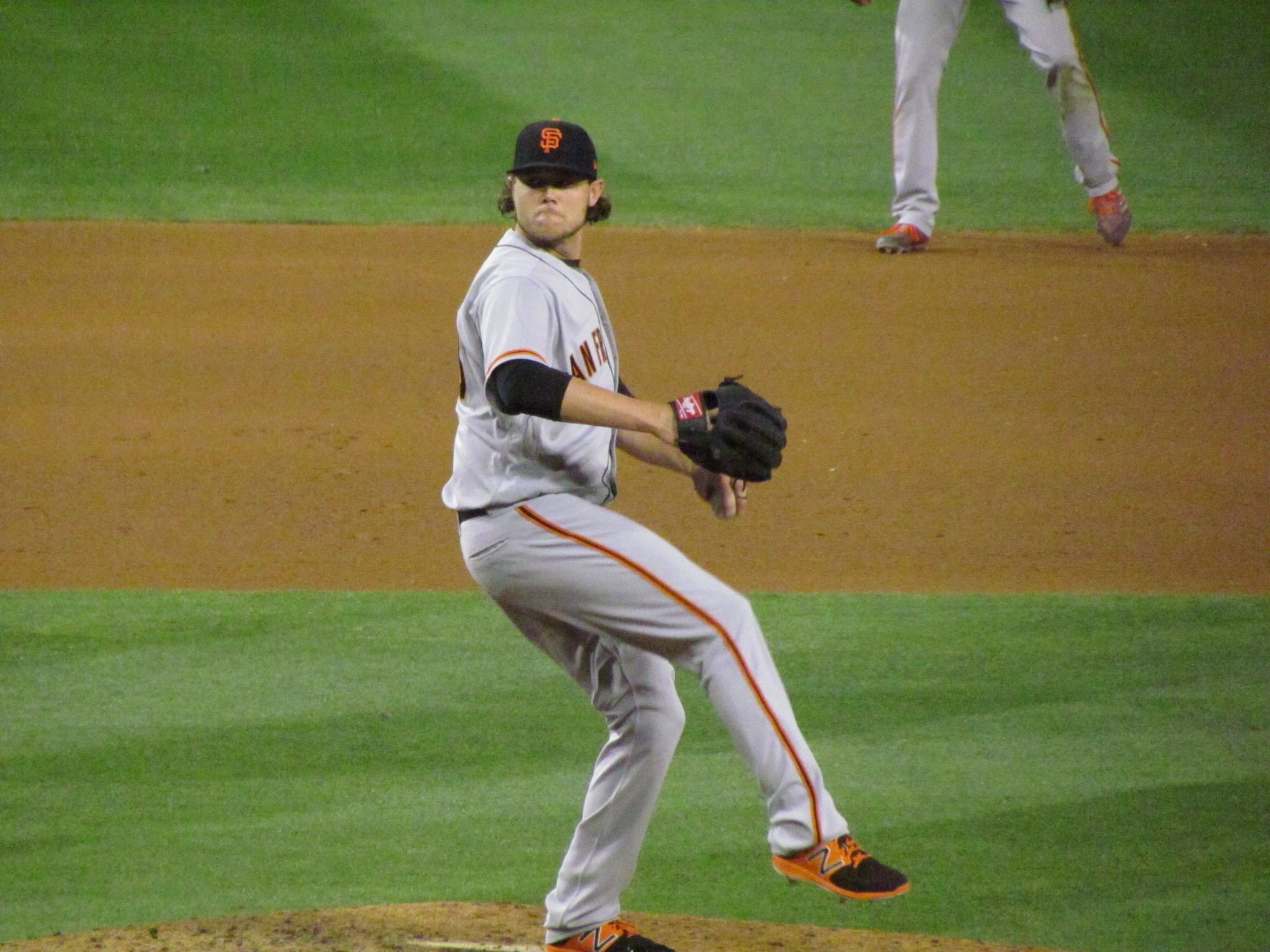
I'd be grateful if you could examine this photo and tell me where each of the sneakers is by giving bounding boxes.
[876,224,927,253]
[1092,185,1132,244]
[547,919,676,952]
[772,834,910,900]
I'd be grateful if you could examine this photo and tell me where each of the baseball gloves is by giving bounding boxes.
[671,380,786,482]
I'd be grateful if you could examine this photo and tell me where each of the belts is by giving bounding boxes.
[457,508,488,525]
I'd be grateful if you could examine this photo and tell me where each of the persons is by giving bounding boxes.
[441,121,909,952]
[851,0,1133,252]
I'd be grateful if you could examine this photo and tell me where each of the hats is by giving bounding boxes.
[506,118,597,179]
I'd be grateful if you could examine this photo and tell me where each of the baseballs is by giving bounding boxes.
[710,490,723,513]
